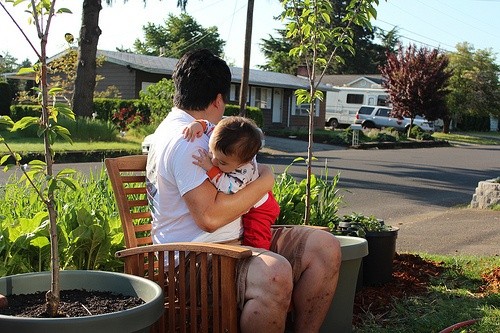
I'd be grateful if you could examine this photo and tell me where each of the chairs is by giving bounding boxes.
[104,154,330,333]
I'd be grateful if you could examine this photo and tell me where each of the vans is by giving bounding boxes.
[325,87,400,130]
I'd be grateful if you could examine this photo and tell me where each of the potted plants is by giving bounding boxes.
[291,0,399,333]
[0,0,165,333]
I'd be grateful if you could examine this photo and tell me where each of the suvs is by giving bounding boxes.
[352,105,435,137]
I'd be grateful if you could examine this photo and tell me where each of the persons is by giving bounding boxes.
[146,48,342,332]
[183,116,279,252]
